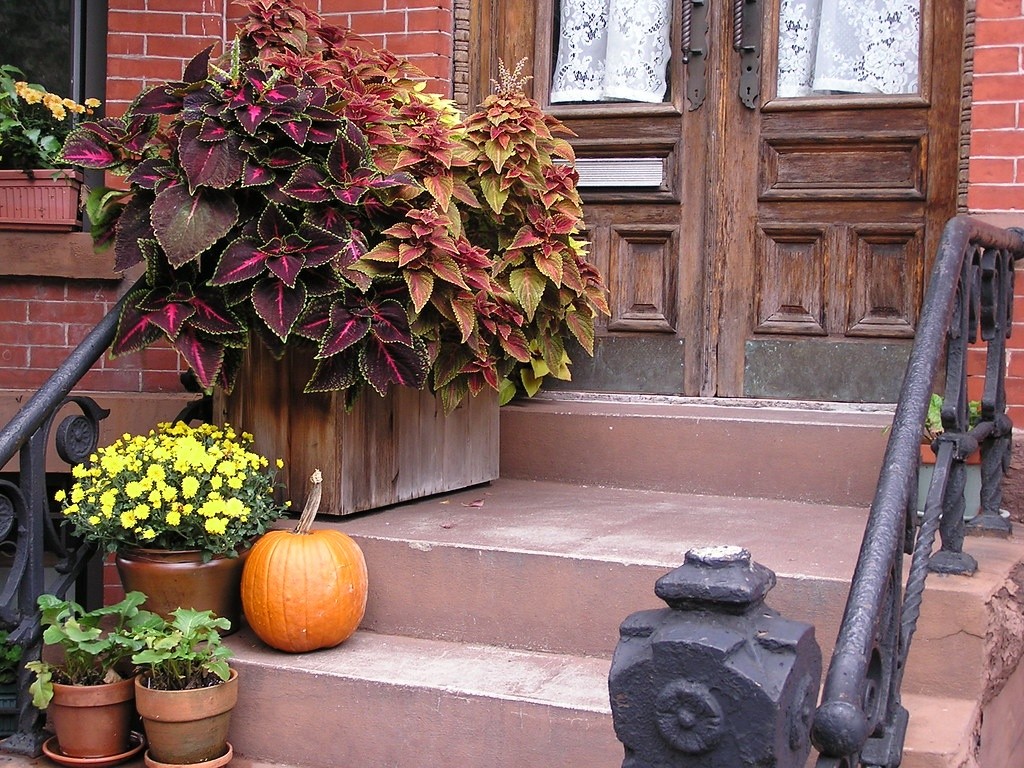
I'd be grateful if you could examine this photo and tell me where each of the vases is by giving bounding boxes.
[0,169,84,227]
[114,534,260,643]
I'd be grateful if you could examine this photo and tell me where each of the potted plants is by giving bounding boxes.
[120,606,239,762]
[883,393,982,520]
[52,0,613,520]
[24,591,161,760]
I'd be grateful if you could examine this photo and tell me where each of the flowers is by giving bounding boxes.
[54,417,294,561]
[0,63,103,177]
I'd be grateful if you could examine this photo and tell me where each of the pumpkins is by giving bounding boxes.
[241,469,368,652]
